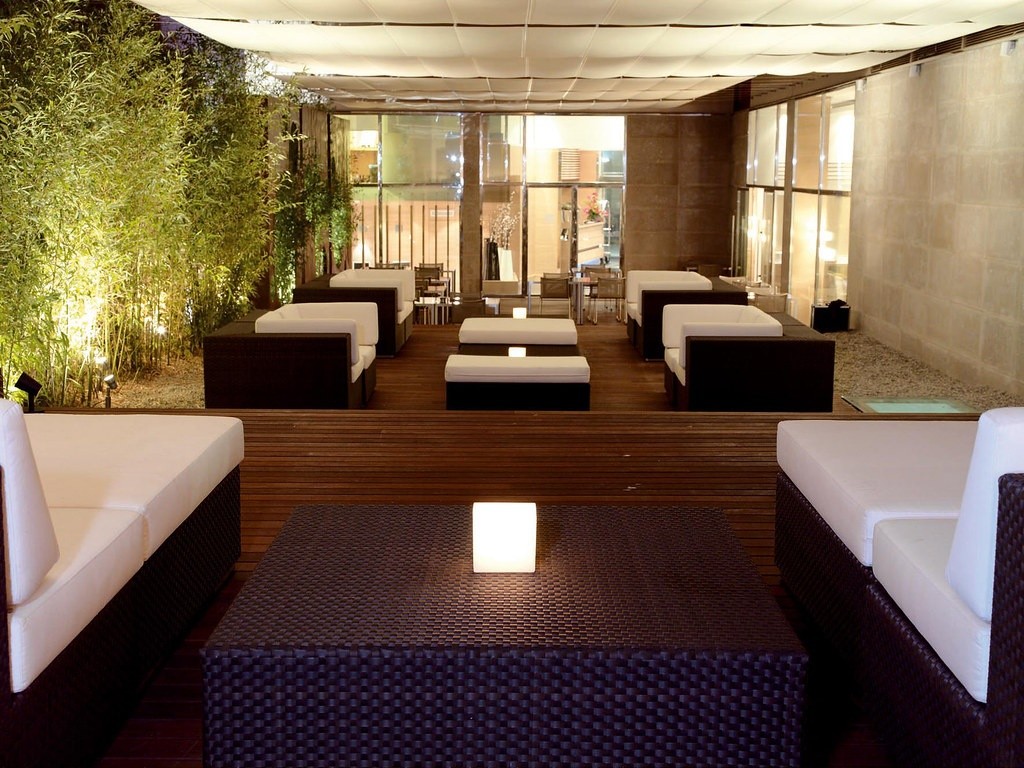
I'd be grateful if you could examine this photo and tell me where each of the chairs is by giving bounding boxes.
[528,264,627,325]
[371,263,450,325]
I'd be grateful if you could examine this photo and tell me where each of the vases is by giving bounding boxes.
[497,247,513,281]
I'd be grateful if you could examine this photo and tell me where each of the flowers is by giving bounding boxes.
[489,190,520,249]
[583,192,609,223]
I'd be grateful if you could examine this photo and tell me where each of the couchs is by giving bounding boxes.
[660,304,836,415]
[624,270,749,362]
[766,404,1024,768]
[293,268,416,355]
[203,300,378,410]
[0,384,247,768]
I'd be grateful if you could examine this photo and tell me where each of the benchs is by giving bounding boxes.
[445,316,591,411]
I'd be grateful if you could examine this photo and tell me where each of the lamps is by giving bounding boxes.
[508,347,527,357]
[513,308,526,319]
[14,370,42,413]
[909,65,921,78]
[471,502,537,574]
[856,79,866,91]
[104,375,117,408]
[999,41,1016,56]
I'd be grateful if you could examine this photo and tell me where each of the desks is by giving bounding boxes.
[459,342,582,358]
[198,499,808,768]
